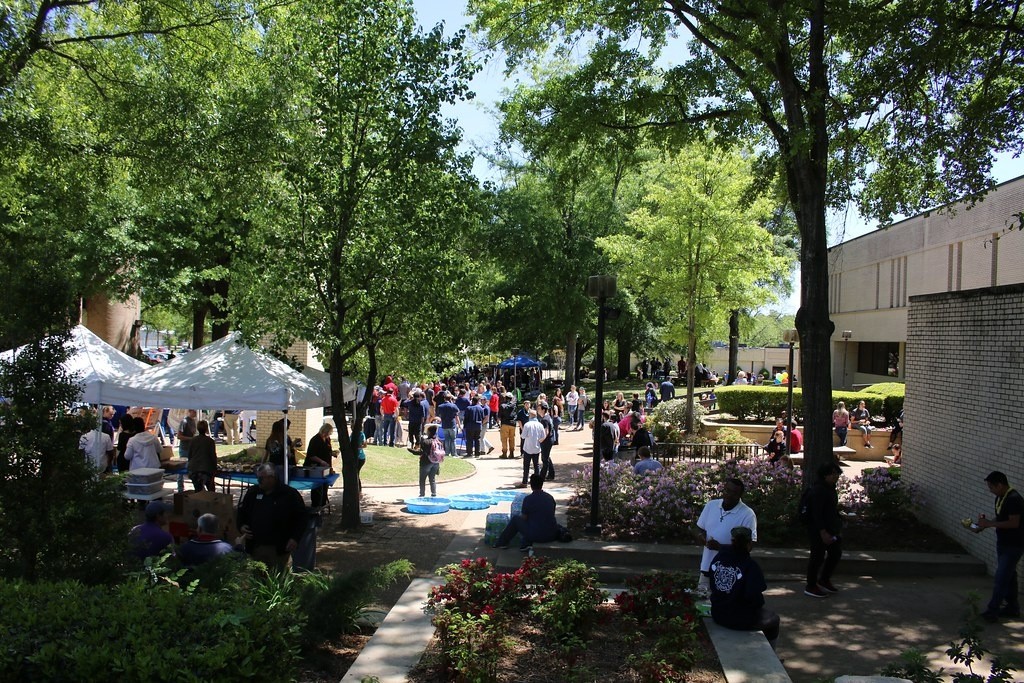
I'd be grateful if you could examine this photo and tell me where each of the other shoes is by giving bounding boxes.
[868,445,874,449]
[365,437,480,459]
[516,482,527,488]
[1001,605,1019,619]
[419,494,424,497]
[487,447,494,454]
[480,451,485,455]
[864,445,868,449]
[981,609,1001,623]
[887,445,893,450]
[431,493,436,496]
[215,439,223,443]
[545,475,554,481]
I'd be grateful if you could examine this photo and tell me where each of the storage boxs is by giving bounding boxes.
[309,467,330,478]
[160,446,174,462]
[126,468,166,495]
[174,490,233,522]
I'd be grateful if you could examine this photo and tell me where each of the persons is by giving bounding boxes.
[766,419,802,467]
[851,401,873,449]
[832,401,850,460]
[700,389,716,411]
[492,474,559,551]
[168,350,175,360]
[588,376,676,461]
[636,356,720,387]
[793,375,797,387]
[803,463,842,598]
[349,364,587,500]
[886,402,904,468]
[694,479,758,598]
[65,405,339,595]
[708,527,780,651]
[724,371,764,385]
[634,446,663,475]
[968,471,1024,624]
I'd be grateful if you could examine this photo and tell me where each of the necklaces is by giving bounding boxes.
[720,509,730,522]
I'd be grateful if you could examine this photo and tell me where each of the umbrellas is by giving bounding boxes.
[497,354,544,368]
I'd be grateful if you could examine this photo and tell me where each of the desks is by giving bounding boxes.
[124,488,174,501]
[159,463,339,516]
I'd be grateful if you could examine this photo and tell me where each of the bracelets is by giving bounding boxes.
[696,532,703,538]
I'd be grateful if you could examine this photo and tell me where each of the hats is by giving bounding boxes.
[731,527,752,543]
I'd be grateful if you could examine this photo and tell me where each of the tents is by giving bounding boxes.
[0,324,358,486]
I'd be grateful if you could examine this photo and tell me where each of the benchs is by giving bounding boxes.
[790,446,857,465]
[701,380,716,387]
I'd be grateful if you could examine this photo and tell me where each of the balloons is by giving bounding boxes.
[775,372,788,384]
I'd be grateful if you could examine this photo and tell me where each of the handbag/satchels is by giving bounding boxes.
[557,522,573,542]
[111,455,119,477]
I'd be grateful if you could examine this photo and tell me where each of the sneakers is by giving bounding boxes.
[519,545,532,552]
[491,544,508,549]
[818,582,837,593]
[804,585,826,597]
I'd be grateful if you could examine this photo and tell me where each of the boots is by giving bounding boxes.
[499,451,507,458]
[509,451,515,458]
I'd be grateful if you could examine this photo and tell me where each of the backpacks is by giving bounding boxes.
[426,438,446,464]
[796,488,814,524]
